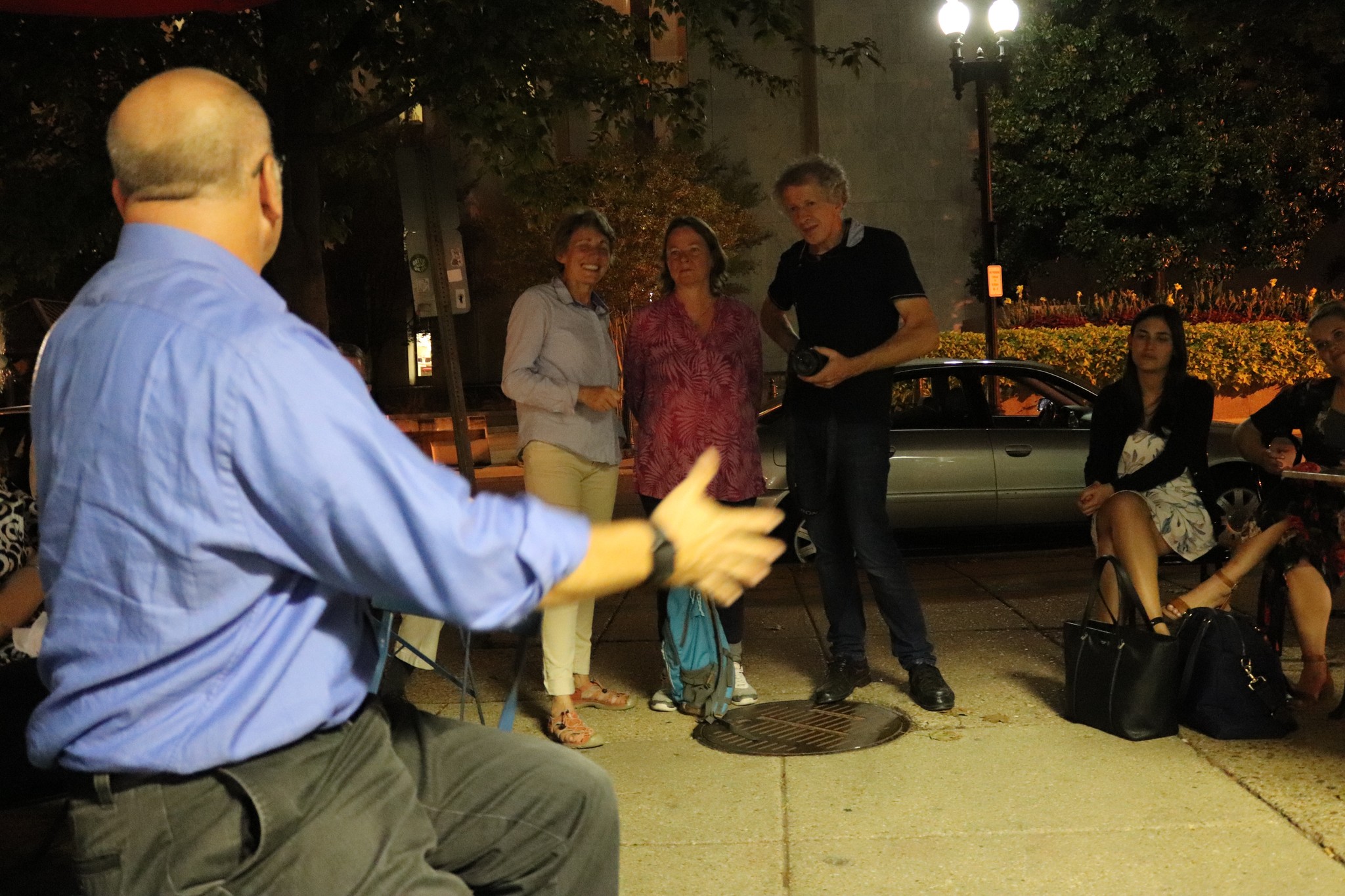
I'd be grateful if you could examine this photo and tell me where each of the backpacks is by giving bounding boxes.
[659,582,760,742]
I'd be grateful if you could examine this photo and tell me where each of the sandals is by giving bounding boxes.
[569,679,639,710]
[548,709,605,750]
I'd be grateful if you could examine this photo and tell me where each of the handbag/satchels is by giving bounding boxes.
[1180,606,1300,741]
[1059,553,1185,742]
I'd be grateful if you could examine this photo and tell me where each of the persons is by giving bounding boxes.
[500,208,630,747]
[1162,297,1345,709]
[623,215,764,711]
[0,312,44,787]
[761,156,954,711]
[1078,303,1222,636]
[334,341,373,393]
[28,67,786,896]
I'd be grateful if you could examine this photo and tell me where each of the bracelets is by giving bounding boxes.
[635,517,675,598]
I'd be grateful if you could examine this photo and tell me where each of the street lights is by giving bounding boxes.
[936,0,1021,412]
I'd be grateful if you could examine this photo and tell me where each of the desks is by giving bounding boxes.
[1281,470,1345,486]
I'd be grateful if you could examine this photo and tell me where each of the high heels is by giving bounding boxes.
[1160,567,1239,623]
[1291,652,1335,709]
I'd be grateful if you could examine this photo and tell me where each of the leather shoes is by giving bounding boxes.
[815,653,871,705]
[908,662,955,711]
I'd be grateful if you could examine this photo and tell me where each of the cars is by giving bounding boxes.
[756,358,1265,561]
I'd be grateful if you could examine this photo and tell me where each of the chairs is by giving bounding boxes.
[1157,500,1231,583]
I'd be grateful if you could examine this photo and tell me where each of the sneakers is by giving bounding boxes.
[650,666,679,711]
[731,660,758,706]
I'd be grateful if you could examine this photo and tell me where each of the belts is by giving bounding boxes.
[55,759,189,800]
